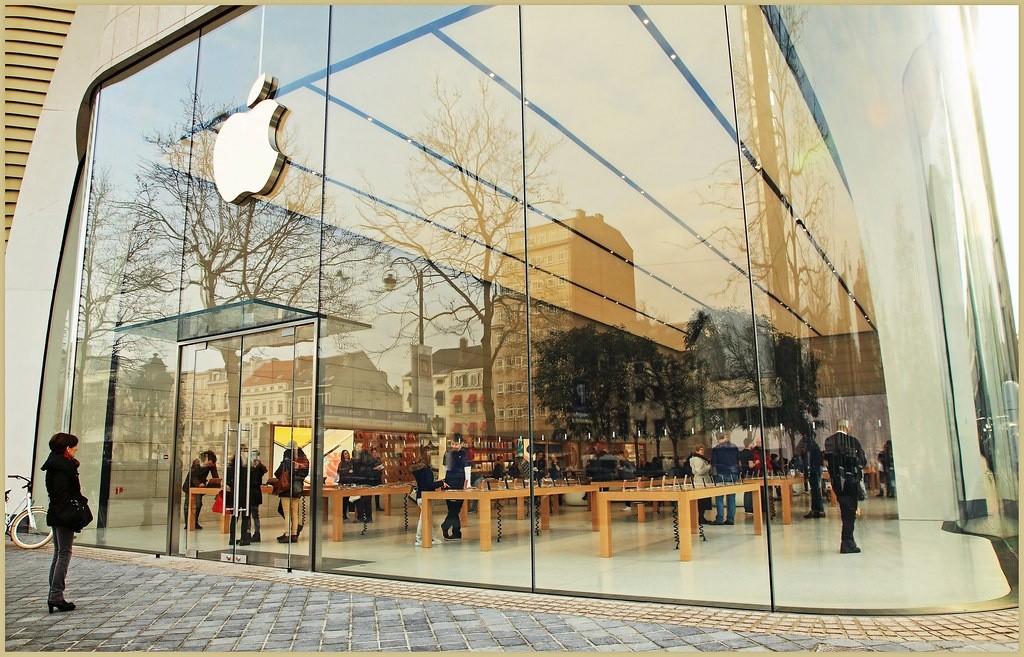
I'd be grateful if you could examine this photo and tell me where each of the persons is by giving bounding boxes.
[796,431,826,517]
[41,433,86,614]
[712,431,738,525]
[741,438,760,514]
[276,440,310,541]
[413,447,442,546]
[337,443,385,521]
[770,454,797,499]
[824,417,867,553]
[225,444,268,544]
[533,452,563,508]
[754,437,773,510]
[580,449,632,511]
[636,455,652,475]
[182,451,221,531]
[494,456,504,480]
[875,441,895,498]
[689,443,713,524]
[442,432,477,541]
[650,455,689,476]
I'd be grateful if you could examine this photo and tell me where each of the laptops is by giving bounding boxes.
[200,478,223,489]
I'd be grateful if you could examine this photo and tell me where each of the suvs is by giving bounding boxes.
[585,453,637,480]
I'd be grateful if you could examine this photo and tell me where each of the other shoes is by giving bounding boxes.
[804,511,824,518]
[280,537,289,543]
[277,534,284,539]
[240,542,249,546]
[841,546,859,553]
[291,535,298,542]
[195,523,202,529]
[701,519,711,524]
[724,521,733,525]
[710,521,723,525]
[376,508,384,511]
[184,524,187,529]
[446,533,461,541]
[620,506,631,511]
[441,524,449,540]
[876,493,883,497]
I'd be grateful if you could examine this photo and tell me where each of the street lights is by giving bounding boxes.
[130,353,175,526]
[382,256,424,345]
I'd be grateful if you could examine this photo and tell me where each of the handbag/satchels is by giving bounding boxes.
[273,461,289,494]
[409,487,417,502]
[54,495,93,532]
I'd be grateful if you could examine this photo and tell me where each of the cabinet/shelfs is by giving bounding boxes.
[354,431,649,487]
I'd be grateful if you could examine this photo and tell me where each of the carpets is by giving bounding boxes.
[175,549,375,571]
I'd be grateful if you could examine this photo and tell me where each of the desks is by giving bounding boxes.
[421,487,601,552]
[298,487,418,541]
[188,487,341,533]
[598,481,763,562]
[384,482,418,516]
[480,467,882,528]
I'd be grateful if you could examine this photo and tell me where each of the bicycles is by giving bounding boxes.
[5,474,54,549]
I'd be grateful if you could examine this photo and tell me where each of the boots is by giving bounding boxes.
[251,532,260,542]
[244,533,251,544]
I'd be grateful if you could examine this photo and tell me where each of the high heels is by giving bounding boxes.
[48,599,75,614]
